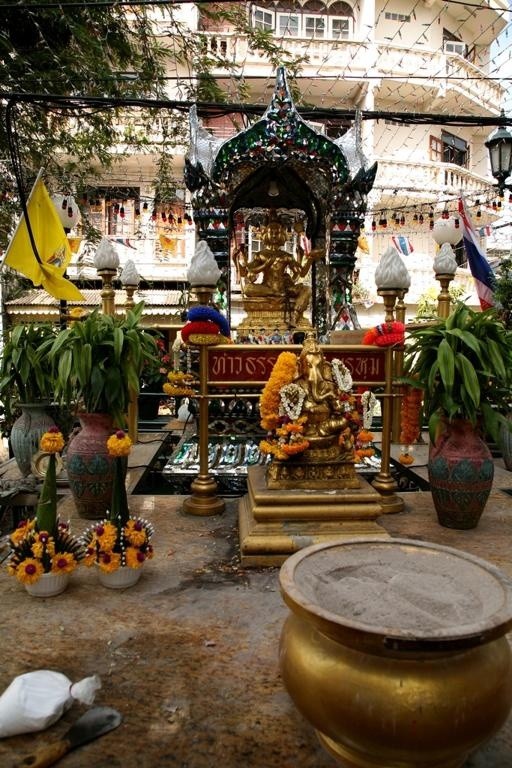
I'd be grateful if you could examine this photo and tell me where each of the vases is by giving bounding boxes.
[20,571,73,597]
[90,559,144,589]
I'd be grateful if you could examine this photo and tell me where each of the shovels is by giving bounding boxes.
[12,706,122,768]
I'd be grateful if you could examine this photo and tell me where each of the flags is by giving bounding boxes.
[478,225,494,237]
[457,198,499,313]
[2,177,87,301]
[392,234,414,256]
[109,238,136,249]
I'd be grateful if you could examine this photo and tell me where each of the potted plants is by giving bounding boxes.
[33,299,164,520]
[0,322,65,477]
[392,294,512,529]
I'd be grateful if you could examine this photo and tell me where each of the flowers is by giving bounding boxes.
[11,426,81,579]
[83,429,153,572]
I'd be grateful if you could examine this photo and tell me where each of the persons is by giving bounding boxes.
[232,218,327,336]
